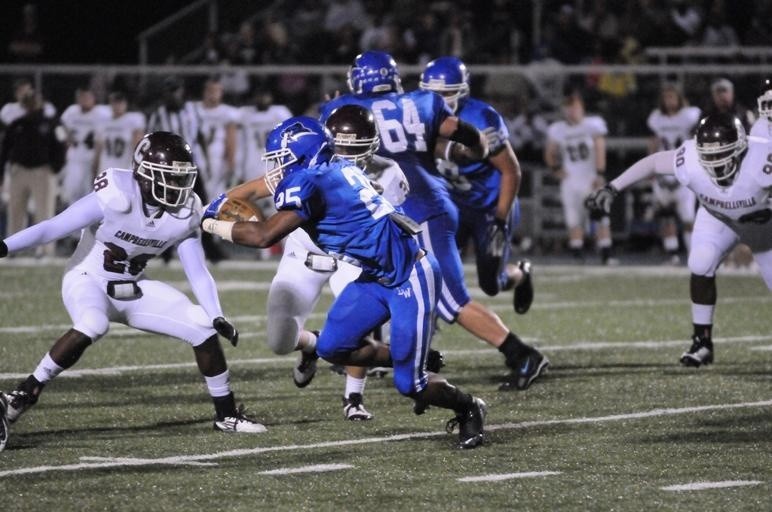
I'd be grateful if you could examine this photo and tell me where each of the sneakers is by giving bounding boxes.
[513,258,534,315]
[292,329,549,449]
[0,391,9,452]
[214,403,268,433]
[4,384,38,423]
[680,333,715,367]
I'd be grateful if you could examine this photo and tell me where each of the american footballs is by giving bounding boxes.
[219,199,265,223]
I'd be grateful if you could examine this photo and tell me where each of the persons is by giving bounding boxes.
[199,115,489,450]
[0,2,772,261]
[0,132,269,433]
[420,56,534,314]
[268,105,410,421]
[586,113,772,364]
[317,50,549,393]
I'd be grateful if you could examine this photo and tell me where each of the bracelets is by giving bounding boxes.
[203,218,237,245]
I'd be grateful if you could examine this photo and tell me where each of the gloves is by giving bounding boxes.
[198,193,227,231]
[479,124,507,155]
[585,184,620,215]
[213,317,238,348]
[480,217,509,262]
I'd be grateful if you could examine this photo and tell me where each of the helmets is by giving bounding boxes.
[132,130,198,208]
[346,49,404,99]
[323,104,381,173]
[695,111,748,189]
[259,116,335,199]
[417,55,473,114]
[755,77,772,123]
